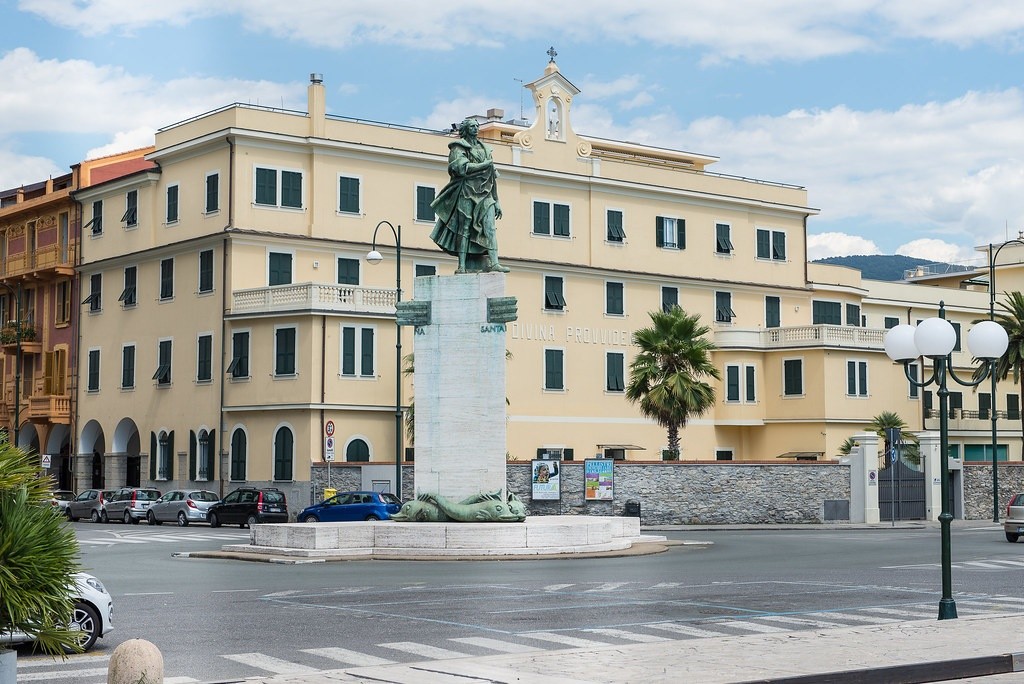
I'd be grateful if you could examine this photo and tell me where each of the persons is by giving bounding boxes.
[534,462,558,483]
[429,118,510,273]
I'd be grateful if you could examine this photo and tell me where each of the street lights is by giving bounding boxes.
[883,320,1010,619]
[366,220,401,501]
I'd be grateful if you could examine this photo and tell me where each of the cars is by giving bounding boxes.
[205,486,288,528]
[65,489,116,522]
[3,575,115,655]
[298,490,402,522]
[102,487,162,524]
[146,489,219,527]
[45,490,76,513]
[1004,491,1024,541]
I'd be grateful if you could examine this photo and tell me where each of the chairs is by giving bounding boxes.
[353,495,362,504]
[243,494,254,502]
